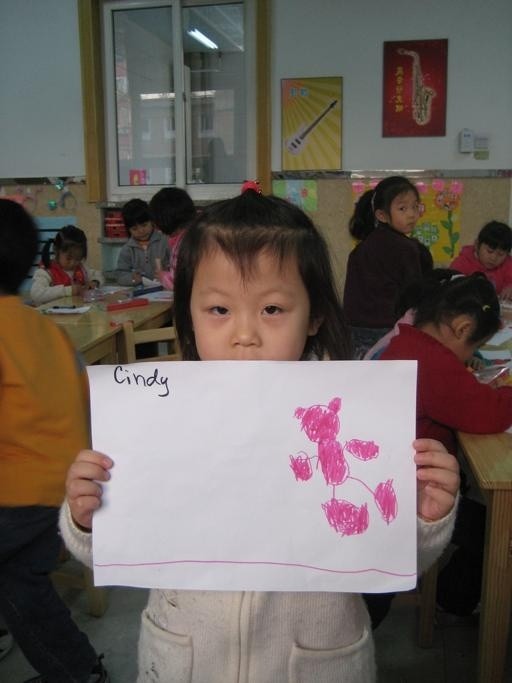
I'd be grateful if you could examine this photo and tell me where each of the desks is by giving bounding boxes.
[415,417,511,683]
[36,278,177,615]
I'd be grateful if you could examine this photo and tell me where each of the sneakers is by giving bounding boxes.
[436,599,472,616]
[1,627,16,660]
[24,653,110,683]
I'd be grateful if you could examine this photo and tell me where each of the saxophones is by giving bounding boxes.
[398,49,435,124]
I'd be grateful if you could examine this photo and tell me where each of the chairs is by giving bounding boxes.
[120,317,184,368]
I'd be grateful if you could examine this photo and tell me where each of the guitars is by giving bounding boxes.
[284,100,340,155]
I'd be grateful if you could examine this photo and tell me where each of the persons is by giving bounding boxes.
[1,192,106,680]
[358,267,485,370]
[60,179,464,681]
[338,172,457,327]
[147,184,202,270]
[366,265,511,459]
[112,197,172,287]
[448,218,511,303]
[24,219,102,305]
